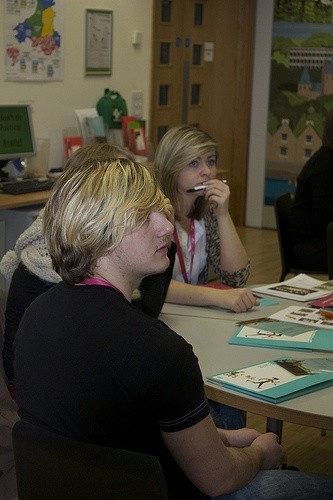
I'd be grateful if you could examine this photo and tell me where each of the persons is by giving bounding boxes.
[287,108,333,273]
[153,125,260,312]
[14,160,333,500]
[0,144,179,407]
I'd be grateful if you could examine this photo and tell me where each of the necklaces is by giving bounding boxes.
[173,215,195,285]
[77,276,127,296]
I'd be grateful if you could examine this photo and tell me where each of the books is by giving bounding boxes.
[226,273,333,352]
[207,355,333,404]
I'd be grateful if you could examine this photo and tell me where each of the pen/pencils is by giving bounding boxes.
[186,179,228,193]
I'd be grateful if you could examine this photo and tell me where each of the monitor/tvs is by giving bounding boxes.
[0,104,38,161]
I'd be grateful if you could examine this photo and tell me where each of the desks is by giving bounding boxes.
[158,284,333,444]
[0,169,230,210]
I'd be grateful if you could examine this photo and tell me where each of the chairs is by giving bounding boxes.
[11,421,170,500]
[273,191,328,281]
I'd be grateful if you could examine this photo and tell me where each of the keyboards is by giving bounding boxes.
[2,179,56,195]
